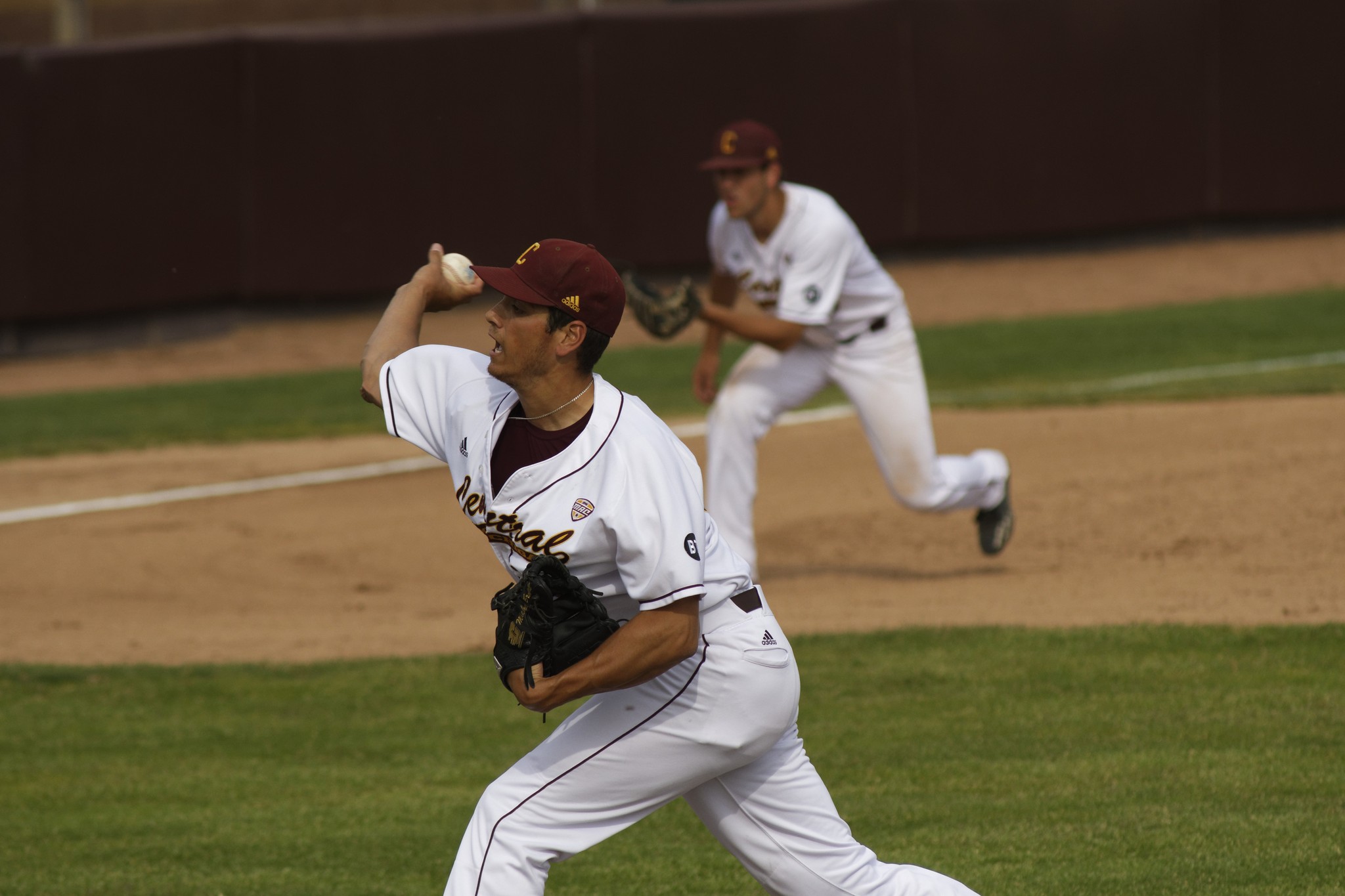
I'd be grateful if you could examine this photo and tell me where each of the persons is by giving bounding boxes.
[693,119,1015,584]
[357,237,981,896]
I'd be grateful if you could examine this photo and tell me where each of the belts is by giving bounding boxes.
[841,317,888,344]
[730,587,764,613]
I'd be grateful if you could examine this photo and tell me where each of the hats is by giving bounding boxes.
[698,120,785,170]
[469,239,625,338]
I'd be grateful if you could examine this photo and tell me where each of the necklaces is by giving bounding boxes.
[507,379,594,419]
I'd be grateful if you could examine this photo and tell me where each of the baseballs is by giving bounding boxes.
[441,252,477,287]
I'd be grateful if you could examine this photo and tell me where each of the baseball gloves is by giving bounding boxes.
[619,270,700,340]
[490,553,622,724]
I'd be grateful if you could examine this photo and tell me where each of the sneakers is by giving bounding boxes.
[976,461,1015,553]
